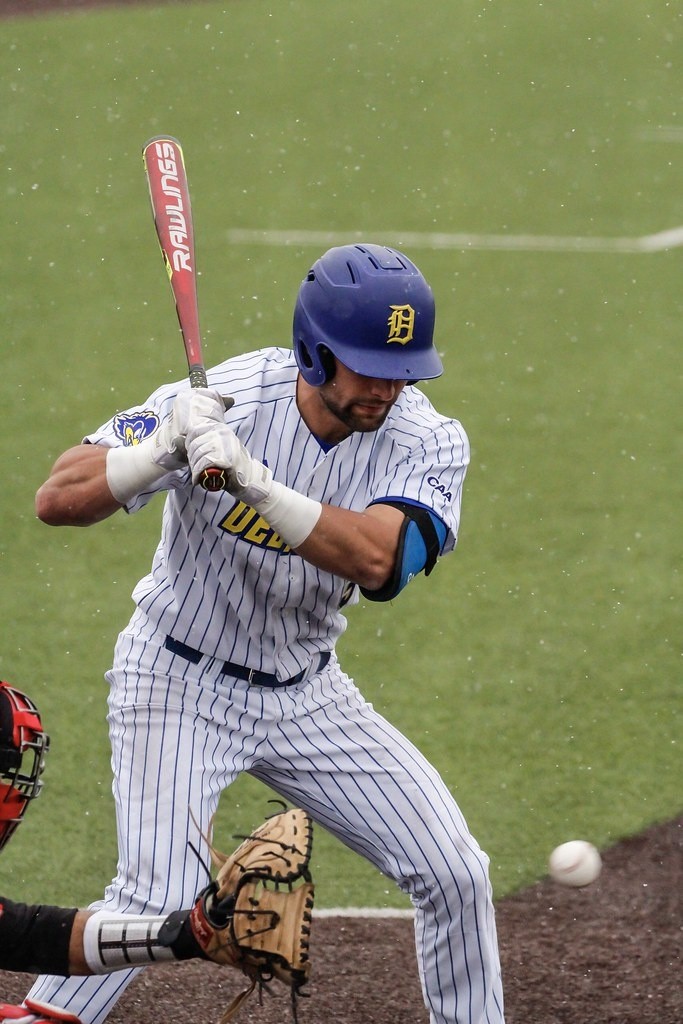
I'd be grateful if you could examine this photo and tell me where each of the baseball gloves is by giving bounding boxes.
[188,805,318,991]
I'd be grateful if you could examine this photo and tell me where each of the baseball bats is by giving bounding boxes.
[140,133,229,493]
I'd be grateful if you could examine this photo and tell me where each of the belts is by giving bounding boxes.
[165,635,331,688]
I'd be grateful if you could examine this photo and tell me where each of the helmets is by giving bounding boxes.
[293,244,444,386]
[0,680,50,852]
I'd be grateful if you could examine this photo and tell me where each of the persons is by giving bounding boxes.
[19,242,505,1024]
[0,679,237,1023]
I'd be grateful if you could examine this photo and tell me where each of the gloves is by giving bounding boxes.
[184,422,272,506]
[150,388,226,471]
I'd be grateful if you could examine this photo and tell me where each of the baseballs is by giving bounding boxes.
[547,838,606,889]
